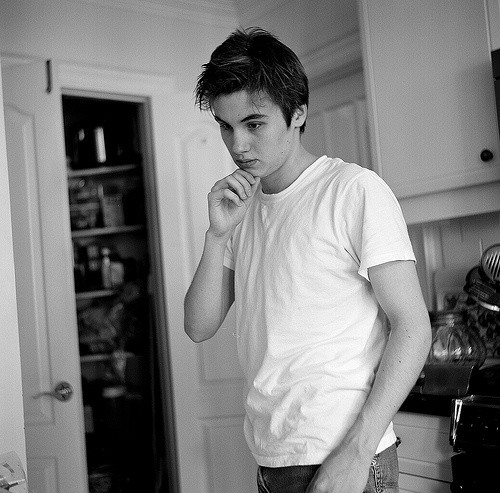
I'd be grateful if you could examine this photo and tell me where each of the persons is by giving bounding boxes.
[183,25,435,493]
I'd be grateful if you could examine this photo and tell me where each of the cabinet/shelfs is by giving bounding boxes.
[356,0,500,225]
[395,412,455,493]
[68,163,156,362]
